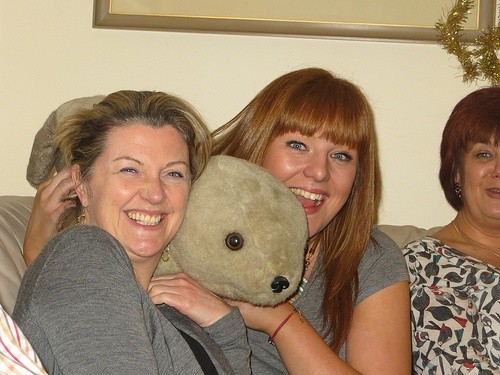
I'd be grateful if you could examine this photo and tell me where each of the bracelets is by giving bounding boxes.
[267,306,304,346]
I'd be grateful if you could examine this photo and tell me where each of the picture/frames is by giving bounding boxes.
[92,0,499,49]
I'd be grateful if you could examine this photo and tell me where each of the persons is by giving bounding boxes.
[11,90,251,375]
[21,66,414,375]
[399,86,499,375]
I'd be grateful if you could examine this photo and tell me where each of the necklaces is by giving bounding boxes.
[289,233,325,300]
[451,221,500,260]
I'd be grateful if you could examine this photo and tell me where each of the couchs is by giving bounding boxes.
[0,194,449,375]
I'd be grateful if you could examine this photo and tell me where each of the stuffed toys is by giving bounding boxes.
[24,95,309,309]
